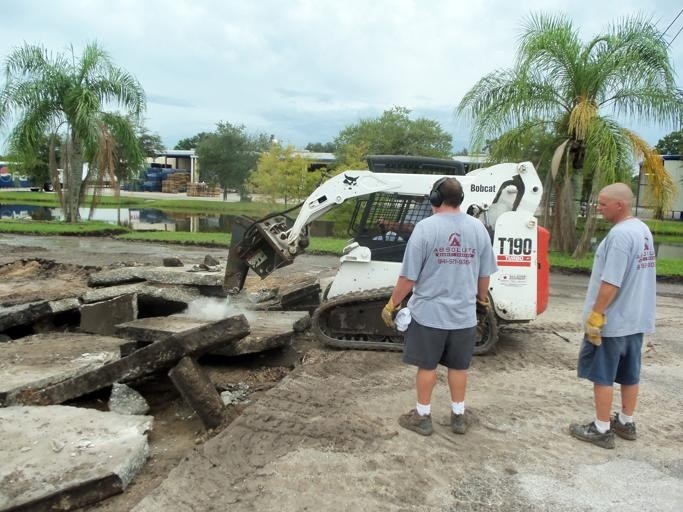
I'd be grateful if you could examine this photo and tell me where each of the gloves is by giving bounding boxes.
[381,297,403,329]
[586,312,605,345]
[476,294,490,315]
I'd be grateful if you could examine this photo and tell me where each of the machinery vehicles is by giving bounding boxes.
[219,152,551,356]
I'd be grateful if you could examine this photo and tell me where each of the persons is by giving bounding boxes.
[381,177,499,437]
[567,181,658,449]
[372,195,435,242]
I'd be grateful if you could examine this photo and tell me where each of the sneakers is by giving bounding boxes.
[450,409,467,433]
[399,408,433,435]
[570,421,615,449]
[610,412,637,440]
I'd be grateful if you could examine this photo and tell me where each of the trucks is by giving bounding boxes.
[0,161,62,192]
